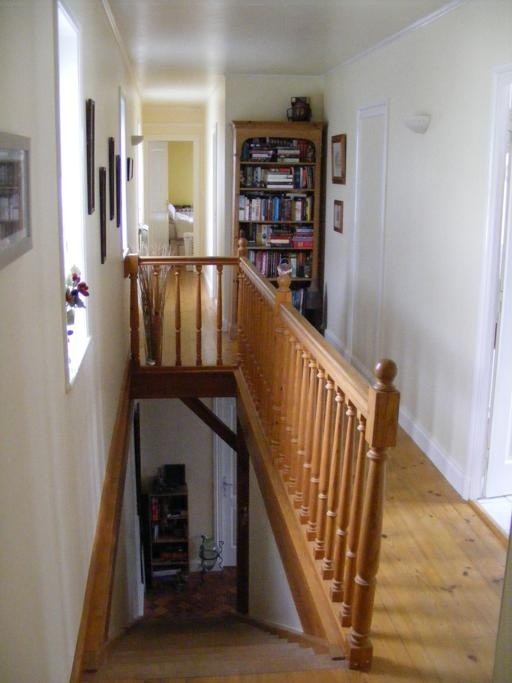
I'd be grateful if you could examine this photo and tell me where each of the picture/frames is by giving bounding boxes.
[0,130,32,268]
[331,135,346,185]
[87,98,133,265]
[333,200,343,234]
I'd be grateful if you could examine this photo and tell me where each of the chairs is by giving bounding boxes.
[168,203,193,239]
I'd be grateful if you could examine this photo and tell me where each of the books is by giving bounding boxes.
[238,137,315,316]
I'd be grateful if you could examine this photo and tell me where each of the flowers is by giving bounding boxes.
[65,260,89,340]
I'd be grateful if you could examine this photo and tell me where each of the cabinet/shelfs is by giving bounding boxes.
[229,121,322,330]
[149,494,188,589]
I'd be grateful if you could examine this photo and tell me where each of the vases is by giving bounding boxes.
[142,292,166,366]
